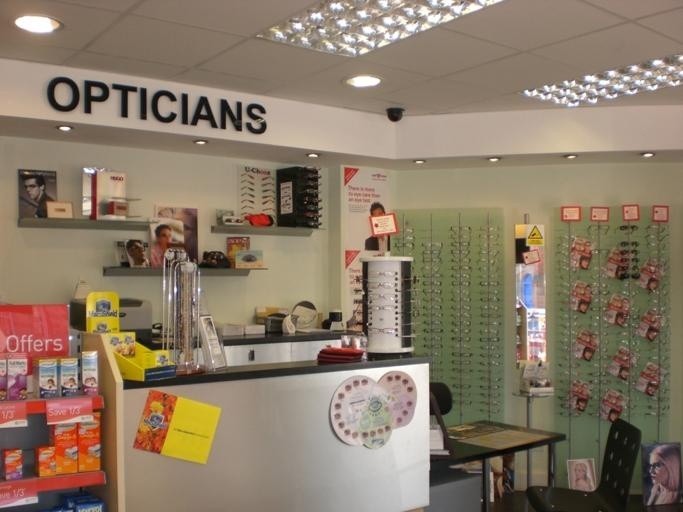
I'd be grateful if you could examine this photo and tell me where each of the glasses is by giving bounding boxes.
[590,372,607,376]
[453,399,471,406]
[592,395,604,400]
[423,257,441,264]
[560,338,574,343]
[590,405,606,409]
[403,289,419,296]
[399,229,413,233]
[480,280,498,286]
[561,314,578,320]
[622,289,639,298]
[422,280,441,286]
[559,380,571,384]
[481,376,500,382]
[425,335,442,341]
[451,336,470,342]
[480,408,499,415]
[620,249,638,255]
[480,241,498,246]
[304,220,322,227]
[556,251,574,256]
[592,282,607,288]
[561,345,574,352]
[452,368,472,373]
[423,329,443,332]
[452,281,470,285]
[649,269,670,289]
[623,282,640,287]
[452,242,470,249]
[431,360,442,364]
[450,265,470,270]
[647,299,669,305]
[451,305,470,309]
[423,313,442,318]
[480,352,499,357]
[593,298,608,304]
[648,355,669,360]
[622,258,639,264]
[481,338,498,342]
[451,297,470,302]
[450,273,470,279]
[590,307,606,311]
[560,329,576,336]
[454,391,470,397]
[453,360,471,366]
[591,387,608,392]
[452,257,470,263]
[423,274,441,279]
[646,241,672,251]
[453,344,470,349]
[424,304,441,310]
[480,360,500,367]
[479,289,497,294]
[592,315,608,320]
[480,274,499,279]
[452,328,470,334]
[557,275,571,279]
[592,331,606,336]
[649,338,670,343]
[560,369,578,379]
[479,233,498,239]
[622,412,635,416]
[23,184,37,189]
[620,241,639,248]
[452,234,470,242]
[369,295,396,300]
[424,288,442,296]
[627,306,638,311]
[303,188,322,195]
[369,270,395,279]
[562,321,577,329]
[618,331,633,336]
[625,405,634,408]
[479,249,497,255]
[425,352,440,356]
[617,340,639,345]
[556,266,576,272]
[647,347,669,352]
[618,264,637,271]
[454,384,470,389]
[481,313,499,319]
[481,345,496,350]
[559,395,573,400]
[562,355,577,359]
[450,249,469,256]
[558,259,577,265]
[366,282,395,292]
[620,387,638,405]
[421,265,442,273]
[559,362,579,368]
[645,393,669,402]
[305,181,322,187]
[646,223,668,234]
[304,212,322,218]
[559,288,573,295]
[645,233,670,243]
[648,255,668,268]
[648,250,669,257]
[596,363,606,369]
[558,233,576,242]
[480,400,500,406]
[421,241,442,249]
[558,243,574,249]
[650,290,669,296]
[591,249,609,257]
[560,387,572,394]
[302,167,318,172]
[481,392,499,398]
[614,225,638,234]
[617,346,640,379]
[645,412,670,419]
[365,327,397,335]
[304,173,321,179]
[408,300,420,307]
[560,403,576,409]
[407,310,420,317]
[596,355,608,360]
[591,291,609,295]
[649,462,662,468]
[424,343,442,349]
[481,321,500,327]
[589,411,603,415]
[307,205,323,211]
[561,298,575,303]
[397,245,413,250]
[648,362,670,393]
[451,227,471,233]
[589,379,608,384]
[560,411,579,417]
[425,320,441,326]
[645,404,668,411]
[482,297,499,302]
[453,352,471,357]
[481,225,497,231]
[451,312,470,319]
[397,236,414,242]
[371,304,395,312]
[651,316,672,338]
[624,314,638,319]
[595,339,609,344]
[587,225,610,234]
[558,280,572,286]
[650,307,669,314]
[452,289,469,295]
[424,250,441,257]
[480,329,498,334]
[591,266,607,271]
[590,323,609,328]
[305,197,321,203]
[592,276,610,279]
[424,296,441,302]
[622,322,638,328]
[479,257,495,263]
[560,306,576,311]
[618,272,639,281]
[625,380,637,386]
[481,385,498,389]
[432,375,443,381]
[480,266,497,271]
[404,276,419,283]
[433,367,442,372]
[452,321,470,326]
[480,305,499,310]
[595,347,607,352]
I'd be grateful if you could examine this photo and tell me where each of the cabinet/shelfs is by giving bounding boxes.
[152,330,362,366]
[0,304,109,511]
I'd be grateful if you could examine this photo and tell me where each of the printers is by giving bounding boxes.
[69,276,153,343]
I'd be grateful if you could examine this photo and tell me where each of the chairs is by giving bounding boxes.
[526,417,643,512]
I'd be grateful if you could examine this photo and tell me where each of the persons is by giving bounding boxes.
[85,376,98,387]
[69,378,77,387]
[46,379,56,390]
[127,239,147,266]
[151,224,174,266]
[361,202,390,252]
[574,464,593,492]
[646,445,680,506]
[22,174,54,218]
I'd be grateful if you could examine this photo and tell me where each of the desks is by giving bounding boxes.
[123,354,434,510]
[443,419,567,510]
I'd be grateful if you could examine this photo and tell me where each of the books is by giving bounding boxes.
[149,218,184,269]
[111,239,149,268]
[640,442,682,506]
[226,236,250,266]
[17,169,57,218]
[234,250,265,269]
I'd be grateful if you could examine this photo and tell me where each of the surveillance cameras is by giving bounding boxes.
[385,104,406,122]
